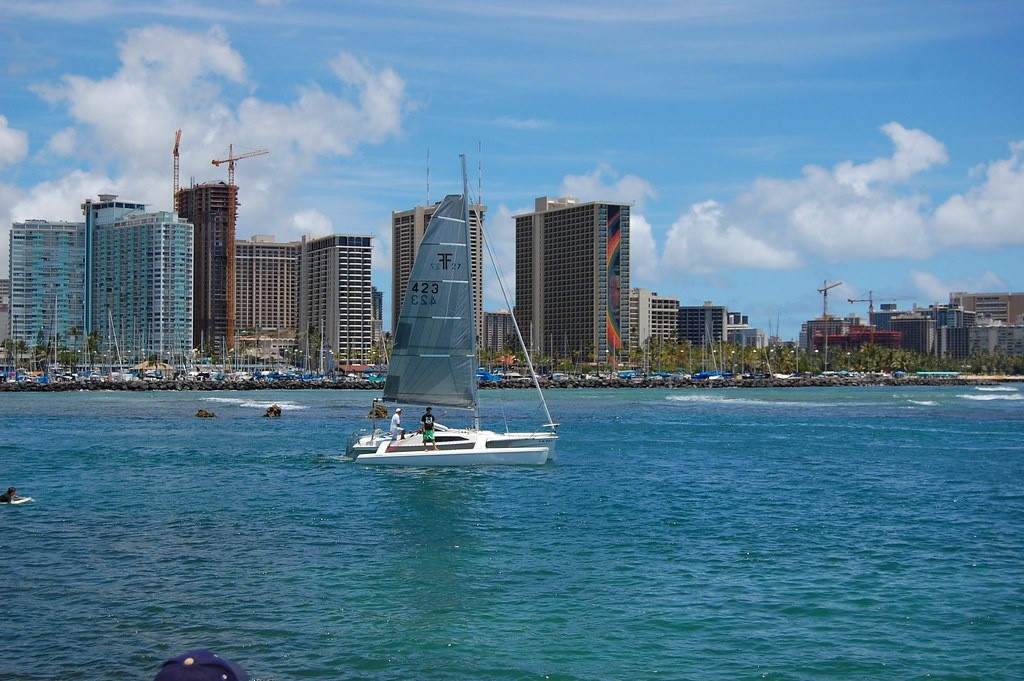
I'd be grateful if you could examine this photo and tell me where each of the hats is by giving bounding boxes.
[395,408,402,413]
[154,649,247,681]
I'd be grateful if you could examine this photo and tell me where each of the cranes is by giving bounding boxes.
[818,279,843,295]
[211,144,268,184]
[172,128,183,192]
[847,290,918,306]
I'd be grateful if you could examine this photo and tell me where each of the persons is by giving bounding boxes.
[0,487,26,504]
[390,408,406,440]
[421,407,440,452]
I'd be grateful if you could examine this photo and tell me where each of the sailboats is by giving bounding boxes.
[1,293,386,391]
[477,321,852,389]
[345,153,558,465]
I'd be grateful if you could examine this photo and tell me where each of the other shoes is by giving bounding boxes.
[401,437,405,439]
[433,446,439,451]
[425,449,428,452]
